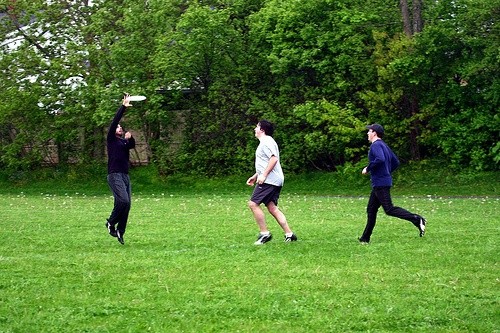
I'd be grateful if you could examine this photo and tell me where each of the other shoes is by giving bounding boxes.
[254,232,273,246]
[284,233,297,243]
[105,221,118,237]
[415,215,426,237]
[115,229,124,244]
[358,237,368,244]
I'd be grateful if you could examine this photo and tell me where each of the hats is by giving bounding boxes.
[366,124,384,136]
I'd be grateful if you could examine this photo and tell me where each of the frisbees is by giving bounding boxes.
[126,96,146,101]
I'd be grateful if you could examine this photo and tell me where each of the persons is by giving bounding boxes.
[246,120,297,245]
[358,124,427,243]
[105,94,135,244]
